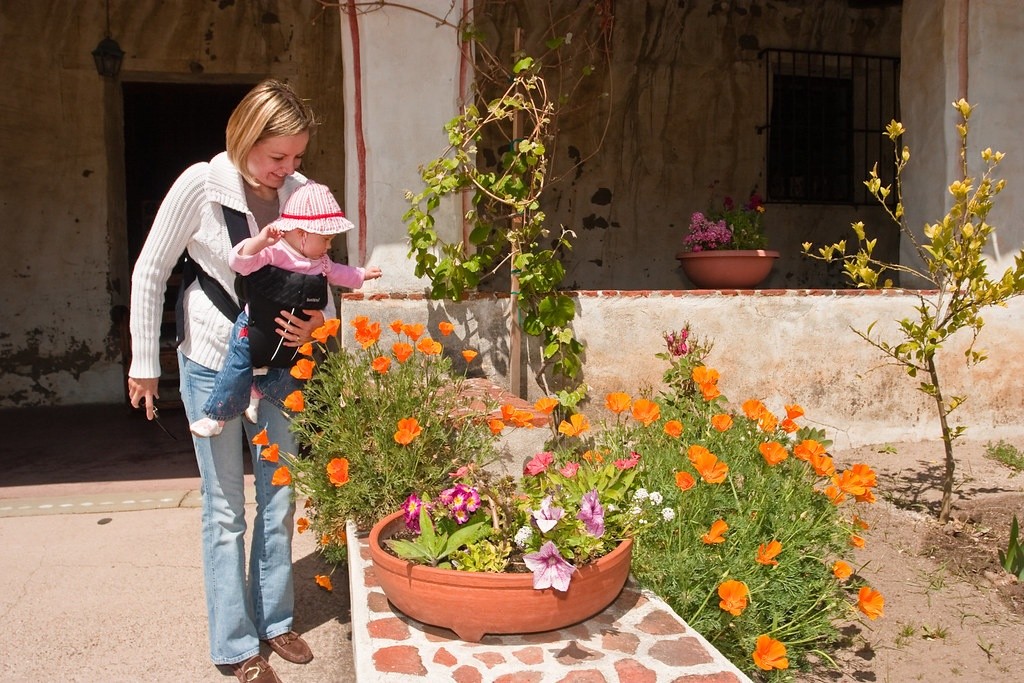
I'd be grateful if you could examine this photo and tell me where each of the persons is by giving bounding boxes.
[127,79,336,683]
[189,181,382,439]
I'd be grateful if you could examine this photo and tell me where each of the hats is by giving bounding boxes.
[269,179,355,235]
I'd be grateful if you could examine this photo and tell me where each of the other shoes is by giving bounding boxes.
[244,396,259,424]
[190,418,223,437]
[262,631,314,664]
[230,654,281,683]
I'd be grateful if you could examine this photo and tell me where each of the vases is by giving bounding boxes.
[370,495,634,642]
[674,250,780,287]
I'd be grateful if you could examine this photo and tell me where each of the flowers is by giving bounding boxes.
[681,206,767,252]
[254,316,882,681]
